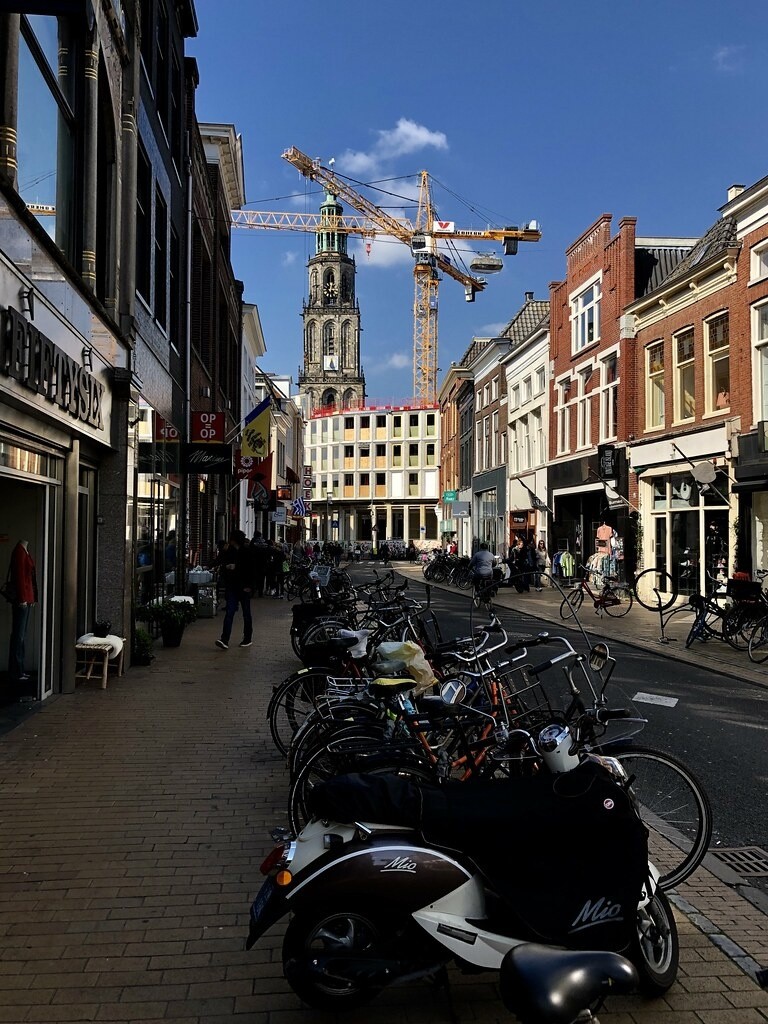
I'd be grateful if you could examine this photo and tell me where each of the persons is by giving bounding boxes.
[501,538,547,593]
[136,529,177,603]
[248,530,285,599]
[467,543,496,604]
[7,539,36,681]
[214,529,259,649]
[292,538,417,566]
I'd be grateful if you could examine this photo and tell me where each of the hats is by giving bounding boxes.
[328,542,333,545]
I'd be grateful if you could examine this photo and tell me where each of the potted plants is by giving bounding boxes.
[93,618,112,638]
[135,600,198,647]
[132,626,151,667]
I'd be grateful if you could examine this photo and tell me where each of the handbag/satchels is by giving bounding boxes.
[283,561,290,572]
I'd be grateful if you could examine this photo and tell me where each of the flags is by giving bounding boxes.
[290,496,305,517]
[245,453,272,506]
[602,482,630,510]
[241,395,270,457]
[519,480,548,512]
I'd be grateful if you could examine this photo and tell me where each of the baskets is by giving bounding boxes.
[311,565,331,586]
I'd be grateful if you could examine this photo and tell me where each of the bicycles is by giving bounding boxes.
[634,567,768,664]
[560,563,632,620]
[265,548,714,894]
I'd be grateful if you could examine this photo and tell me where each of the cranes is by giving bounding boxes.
[230,144,543,407]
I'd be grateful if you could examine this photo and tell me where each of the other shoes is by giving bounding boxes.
[535,588,539,592]
[270,589,275,596]
[280,595,284,599]
[265,590,270,595]
[278,596,281,599]
[539,588,542,592]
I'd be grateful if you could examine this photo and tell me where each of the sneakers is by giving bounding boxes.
[215,639,229,650]
[239,638,253,647]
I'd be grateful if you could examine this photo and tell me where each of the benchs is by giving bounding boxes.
[75,637,127,689]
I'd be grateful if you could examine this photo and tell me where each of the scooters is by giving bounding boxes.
[244,644,679,1013]
